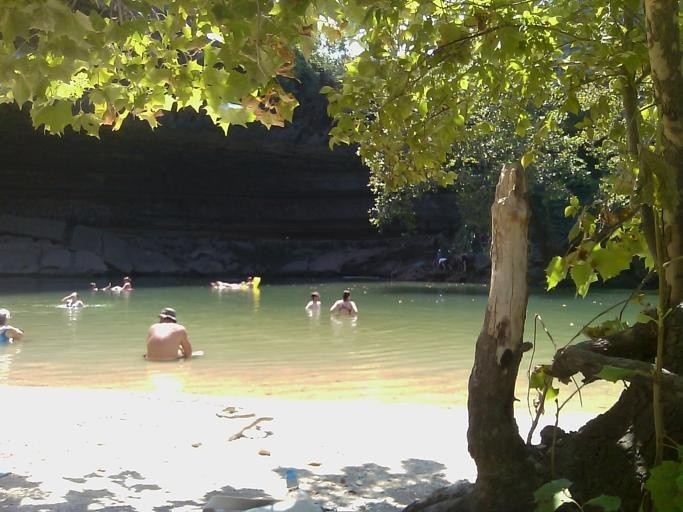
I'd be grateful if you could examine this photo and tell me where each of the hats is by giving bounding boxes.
[157,307,177,322]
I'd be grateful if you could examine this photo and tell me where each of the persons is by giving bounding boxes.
[460,247,475,274]
[110,276,131,291]
[0,306,25,347]
[305,290,321,311]
[89,281,110,291]
[209,275,253,291]
[143,306,192,362]
[328,289,359,316]
[435,247,452,272]
[60,291,83,308]
